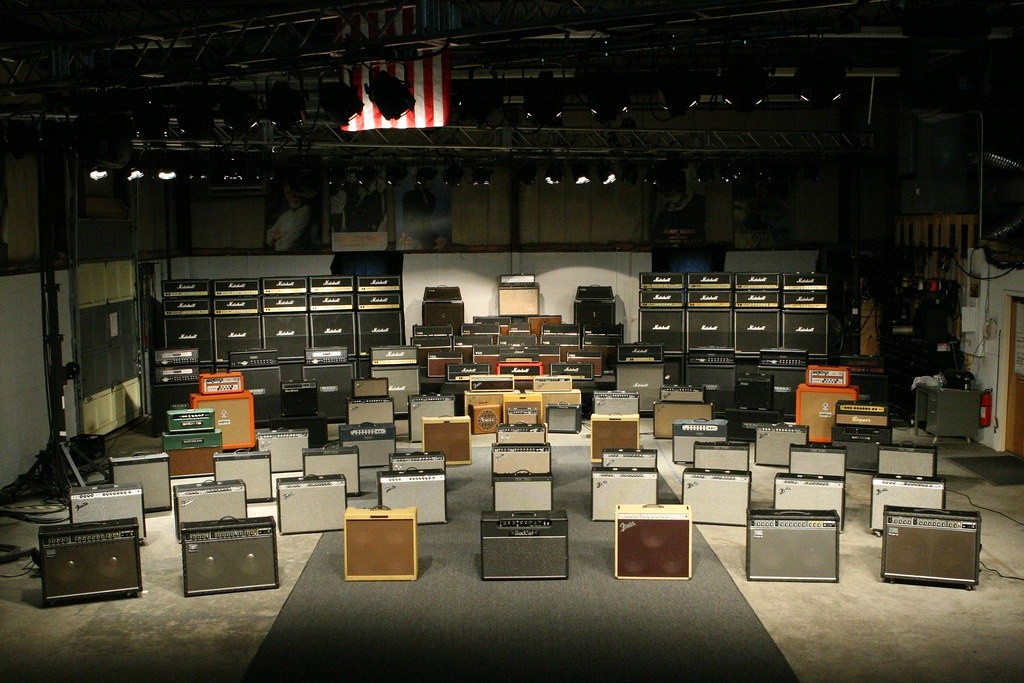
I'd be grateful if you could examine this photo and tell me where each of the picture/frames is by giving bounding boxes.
[646,161,708,250]
[732,163,791,251]
[393,166,453,254]
[263,171,325,253]
[328,158,388,252]
